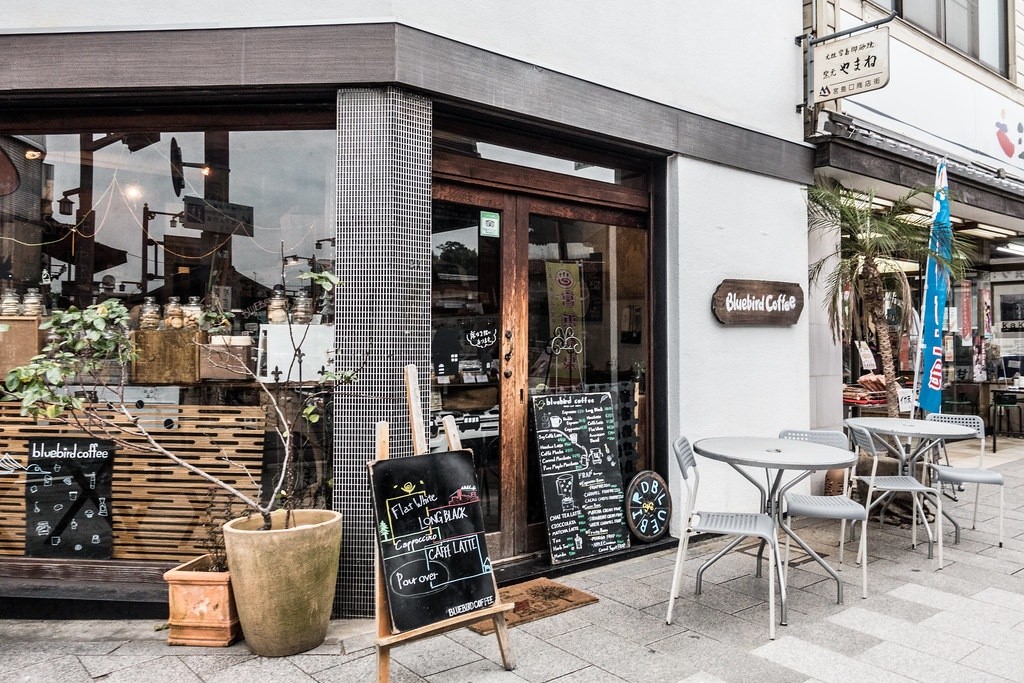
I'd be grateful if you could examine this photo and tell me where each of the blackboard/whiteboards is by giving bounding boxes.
[531,392,632,566]
[367,448,498,636]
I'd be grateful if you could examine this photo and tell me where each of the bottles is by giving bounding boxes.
[292,291,313,324]
[0,288,20,315]
[139,297,161,331]
[164,296,184,329]
[267,290,288,325]
[180,296,204,329]
[22,287,43,316]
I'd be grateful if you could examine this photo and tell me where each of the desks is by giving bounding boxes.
[693,435,857,627]
[952,379,1014,437]
[991,388,1024,454]
[843,417,976,559]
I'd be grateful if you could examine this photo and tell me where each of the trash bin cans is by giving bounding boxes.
[842,403,862,453]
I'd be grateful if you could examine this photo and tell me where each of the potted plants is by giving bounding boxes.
[801,171,981,508]
[163,466,255,647]
[1,251,369,658]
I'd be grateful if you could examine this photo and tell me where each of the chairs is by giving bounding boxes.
[777,429,868,603]
[850,425,944,570]
[666,435,781,640]
[919,412,1005,550]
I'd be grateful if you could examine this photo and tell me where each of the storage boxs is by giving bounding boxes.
[128,329,204,384]
[198,345,255,380]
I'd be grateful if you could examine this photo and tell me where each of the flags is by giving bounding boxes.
[916,163,952,416]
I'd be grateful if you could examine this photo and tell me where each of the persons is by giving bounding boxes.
[97,275,116,306]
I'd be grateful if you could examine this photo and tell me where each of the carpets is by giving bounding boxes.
[465,575,600,637]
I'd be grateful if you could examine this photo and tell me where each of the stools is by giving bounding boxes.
[941,401,977,424]
[997,402,1023,438]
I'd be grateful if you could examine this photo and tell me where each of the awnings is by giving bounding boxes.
[42,218,128,274]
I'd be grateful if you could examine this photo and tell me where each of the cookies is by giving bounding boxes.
[267,301,287,322]
[138,307,202,330]
[0,300,42,315]
